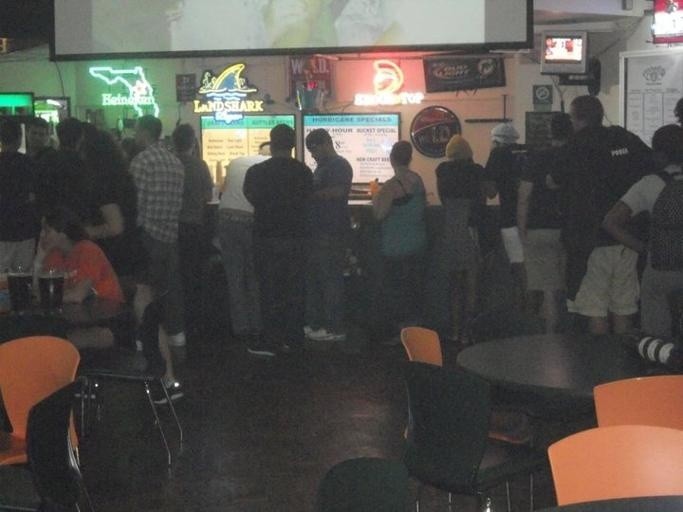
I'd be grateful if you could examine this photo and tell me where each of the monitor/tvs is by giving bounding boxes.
[540,31,588,75]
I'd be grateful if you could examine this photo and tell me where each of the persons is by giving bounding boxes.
[515,112,577,336]
[433,135,485,345]
[25,117,59,211]
[299,127,354,345]
[77,129,185,406]
[0,114,39,277]
[39,206,126,371]
[370,140,431,348]
[56,117,82,167]
[543,94,652,336]
[243,123,313,356]
[218,143,273,342]
[598,123,683,340]
[169,123,213,359]
[126,113,188,353]
[483,123,536,294]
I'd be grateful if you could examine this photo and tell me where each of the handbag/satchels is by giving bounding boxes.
[380,179,425,256]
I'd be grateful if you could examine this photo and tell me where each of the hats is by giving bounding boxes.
[445,134,473,158]
[491,123,520,145]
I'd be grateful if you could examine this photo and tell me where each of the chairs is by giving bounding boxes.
[400,326,444,368]
[315,456,414,512]
[455,332,647,392]
[1,336,81,468]
[547,425,683,505]
[399,359,545,511]
[544,496,683,512]
[593,375,682,429]
[0,379,85,512]
[82,289,183,465]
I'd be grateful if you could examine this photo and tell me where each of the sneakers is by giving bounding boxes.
[166,331,186,347]
[378,334,402,346]
[307,327,346,341]
[246,341,276,356]
[304,324,317,338]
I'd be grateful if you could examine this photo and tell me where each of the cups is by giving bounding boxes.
[7,273,34,317]
[39,274,64,317]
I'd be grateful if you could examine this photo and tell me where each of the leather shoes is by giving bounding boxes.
[154,381,182,404]
